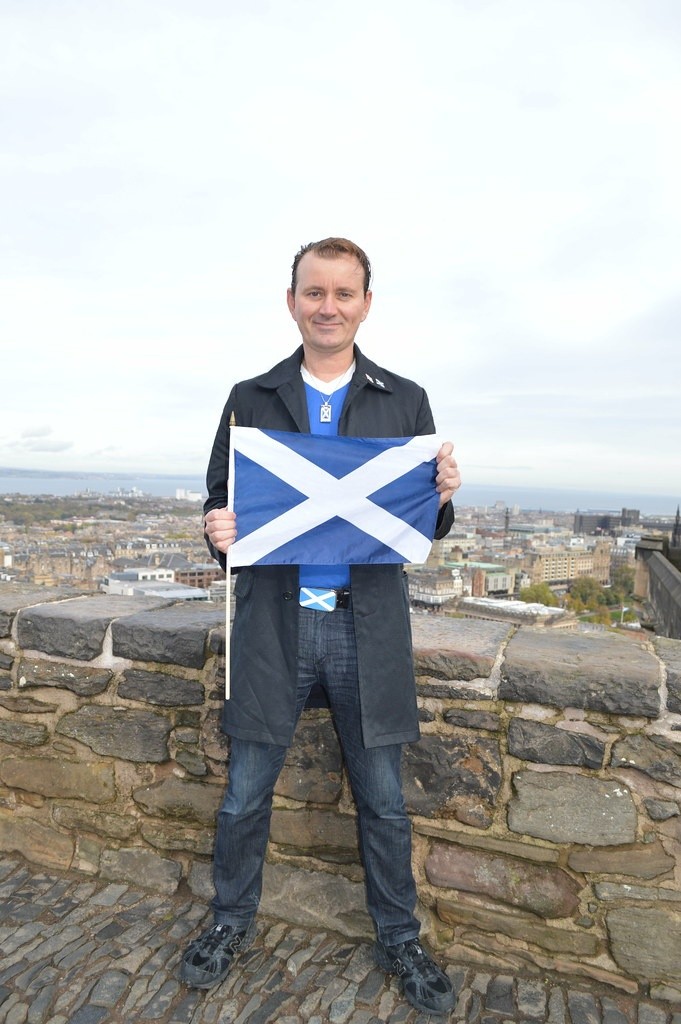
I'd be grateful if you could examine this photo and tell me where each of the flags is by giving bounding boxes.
[226,426,444,567]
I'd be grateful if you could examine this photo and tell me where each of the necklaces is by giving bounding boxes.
[314,373,346,422]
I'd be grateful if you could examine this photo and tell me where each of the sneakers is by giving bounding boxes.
[181,918,258,989]
[373,936,458,1016]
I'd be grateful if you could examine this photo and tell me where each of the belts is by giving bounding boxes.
[298,587,350,613]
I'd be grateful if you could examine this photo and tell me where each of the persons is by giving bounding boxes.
[182,237,461,1015]
